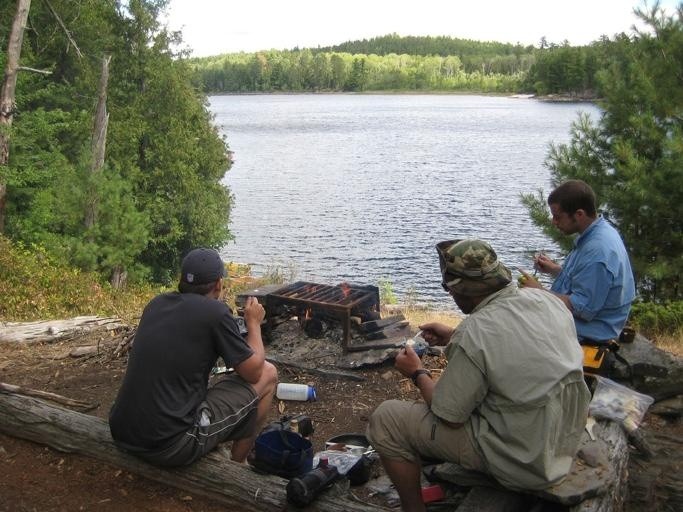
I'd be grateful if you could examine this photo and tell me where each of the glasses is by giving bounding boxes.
[440,277,461,292]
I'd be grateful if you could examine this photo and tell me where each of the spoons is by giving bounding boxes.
[406,330,425,346]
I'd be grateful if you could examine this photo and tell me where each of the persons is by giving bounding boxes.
[107,244,280,466]
[514,177,635,347]
[363,236,593,512]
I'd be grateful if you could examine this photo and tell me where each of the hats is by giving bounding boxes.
[435,238,512,296]
[179,247,227,285]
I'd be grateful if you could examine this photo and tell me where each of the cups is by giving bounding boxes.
[400,338,429,360]
[276,382,317,402]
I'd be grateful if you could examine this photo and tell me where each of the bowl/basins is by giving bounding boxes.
[326,433,370,451]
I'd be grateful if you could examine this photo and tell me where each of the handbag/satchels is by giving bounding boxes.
[251,428,313,478]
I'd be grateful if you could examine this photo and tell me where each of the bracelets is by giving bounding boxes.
[410,369,432,388]
